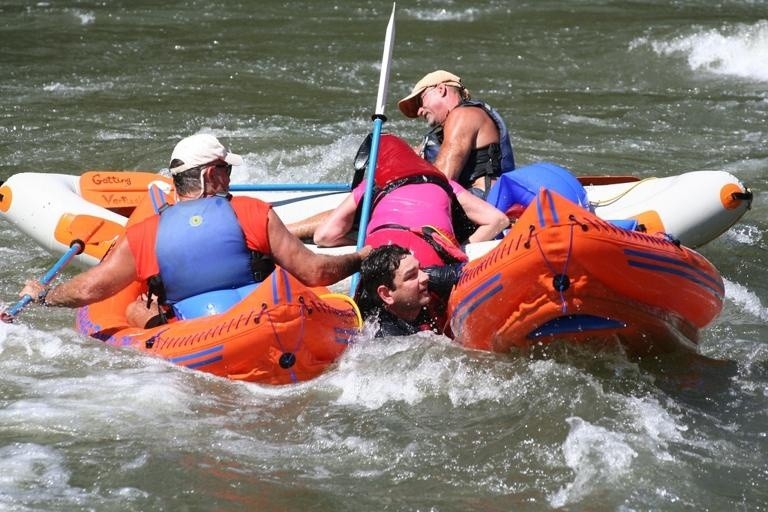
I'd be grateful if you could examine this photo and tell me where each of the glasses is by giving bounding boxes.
[214,163,232,176]
[416,88,436,106]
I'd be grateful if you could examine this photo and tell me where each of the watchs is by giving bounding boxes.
[38,288,51,307]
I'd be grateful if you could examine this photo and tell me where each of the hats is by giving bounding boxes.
[170,134,242,176]
[398,70,471,117]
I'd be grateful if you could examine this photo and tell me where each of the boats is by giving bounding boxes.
[1,170,750,290]
[71,263,363,387]
[445,187,727,353]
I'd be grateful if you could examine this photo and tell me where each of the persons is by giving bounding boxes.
[19,134,375,330]
[313,132,511,324]
[283,70,516,244]
[357,243,468,339]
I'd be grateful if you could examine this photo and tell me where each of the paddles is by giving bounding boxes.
[2,214,124,319]
[81,170,353,208]
[350,2,396,297]
